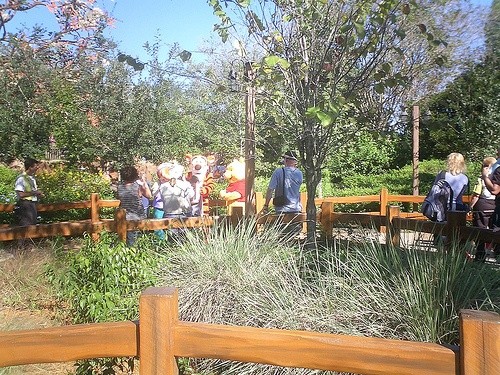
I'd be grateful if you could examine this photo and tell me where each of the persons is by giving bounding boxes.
[433,152,470,248]
[482,165,500,264]
[104,156,202,246]
[262,151,303,216]
[14,156,44,225]
[468,157,497,258]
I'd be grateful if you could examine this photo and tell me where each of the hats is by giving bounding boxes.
[159,161,186,183]
[281,151,298,160]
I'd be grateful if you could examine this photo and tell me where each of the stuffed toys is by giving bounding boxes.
[183,153,214,218]
[220,159,248,216]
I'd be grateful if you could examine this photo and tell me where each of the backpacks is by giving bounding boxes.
[421,178,453,223]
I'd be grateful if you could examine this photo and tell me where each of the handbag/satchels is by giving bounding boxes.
[271,194,290,207]
